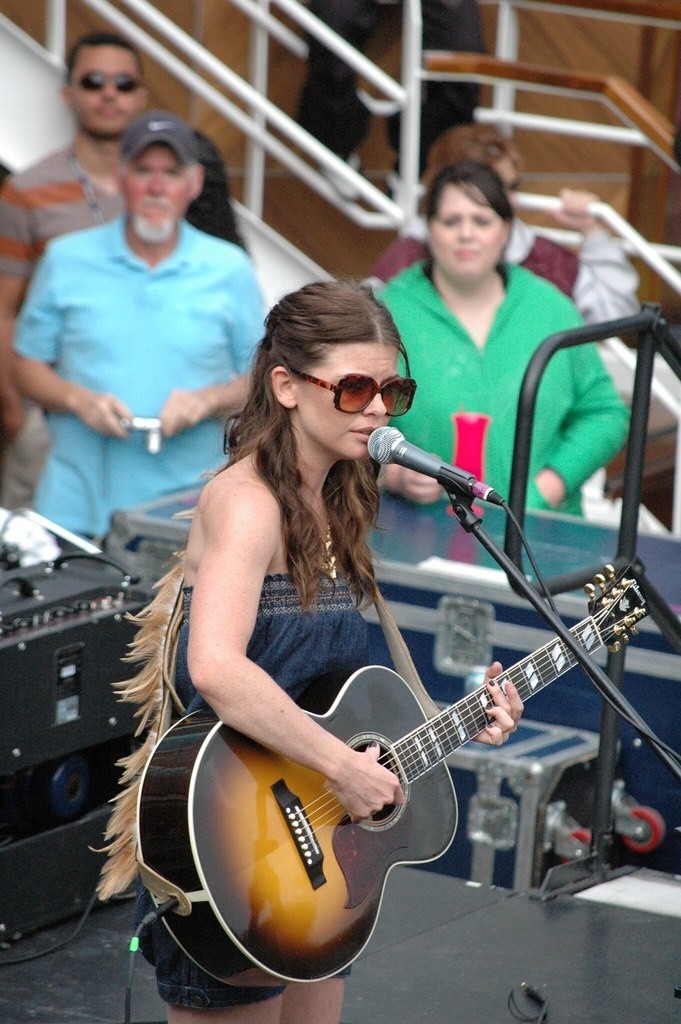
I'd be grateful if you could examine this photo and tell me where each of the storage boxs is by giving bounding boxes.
[108,477,681,876]
[406,693,624,891]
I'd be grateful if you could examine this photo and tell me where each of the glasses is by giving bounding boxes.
[72,71,143,91]
[288,369,417,416]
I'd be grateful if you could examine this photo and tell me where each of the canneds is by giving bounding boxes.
[463,666,487,695]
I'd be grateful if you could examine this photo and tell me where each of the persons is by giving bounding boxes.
[86,274,523,1024]
[1,29,268,551]
[361,126,643,518]
[290,1,483,199]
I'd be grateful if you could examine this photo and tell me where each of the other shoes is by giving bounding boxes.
[319,154,363,202]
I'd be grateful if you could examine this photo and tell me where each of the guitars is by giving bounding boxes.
[134,557,658,988]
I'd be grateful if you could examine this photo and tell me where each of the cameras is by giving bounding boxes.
[122,416,165,454]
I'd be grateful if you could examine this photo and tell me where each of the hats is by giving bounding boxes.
[117,112,199,164]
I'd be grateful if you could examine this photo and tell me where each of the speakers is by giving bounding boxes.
[0,550,155,774]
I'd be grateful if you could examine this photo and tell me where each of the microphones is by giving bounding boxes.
[368,426,506,506]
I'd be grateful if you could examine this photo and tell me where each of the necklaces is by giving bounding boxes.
[318,522,337,580]
